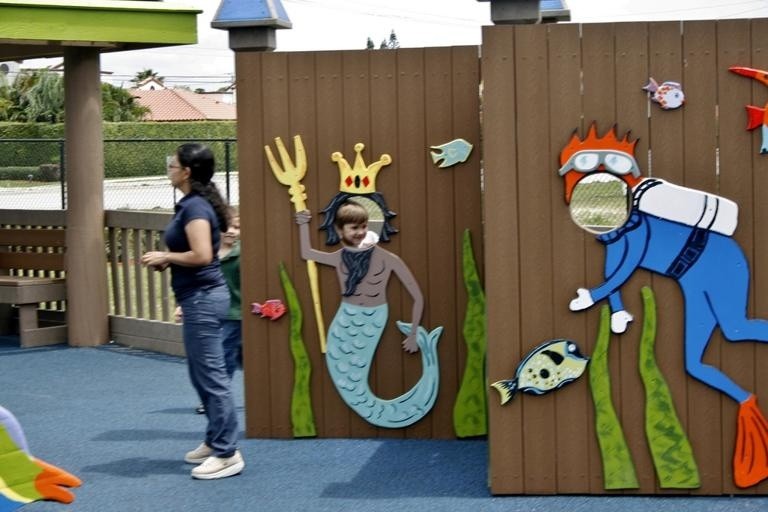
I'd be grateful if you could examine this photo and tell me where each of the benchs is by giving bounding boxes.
[0,224,69,350]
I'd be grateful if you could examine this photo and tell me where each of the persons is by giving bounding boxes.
[139,142,247,481]
[170,203,242,412]
[336,198,369,246]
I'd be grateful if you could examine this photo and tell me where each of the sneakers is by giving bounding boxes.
[185,439,217,464]
[190,448,246,480]
[196,401,206,414]
